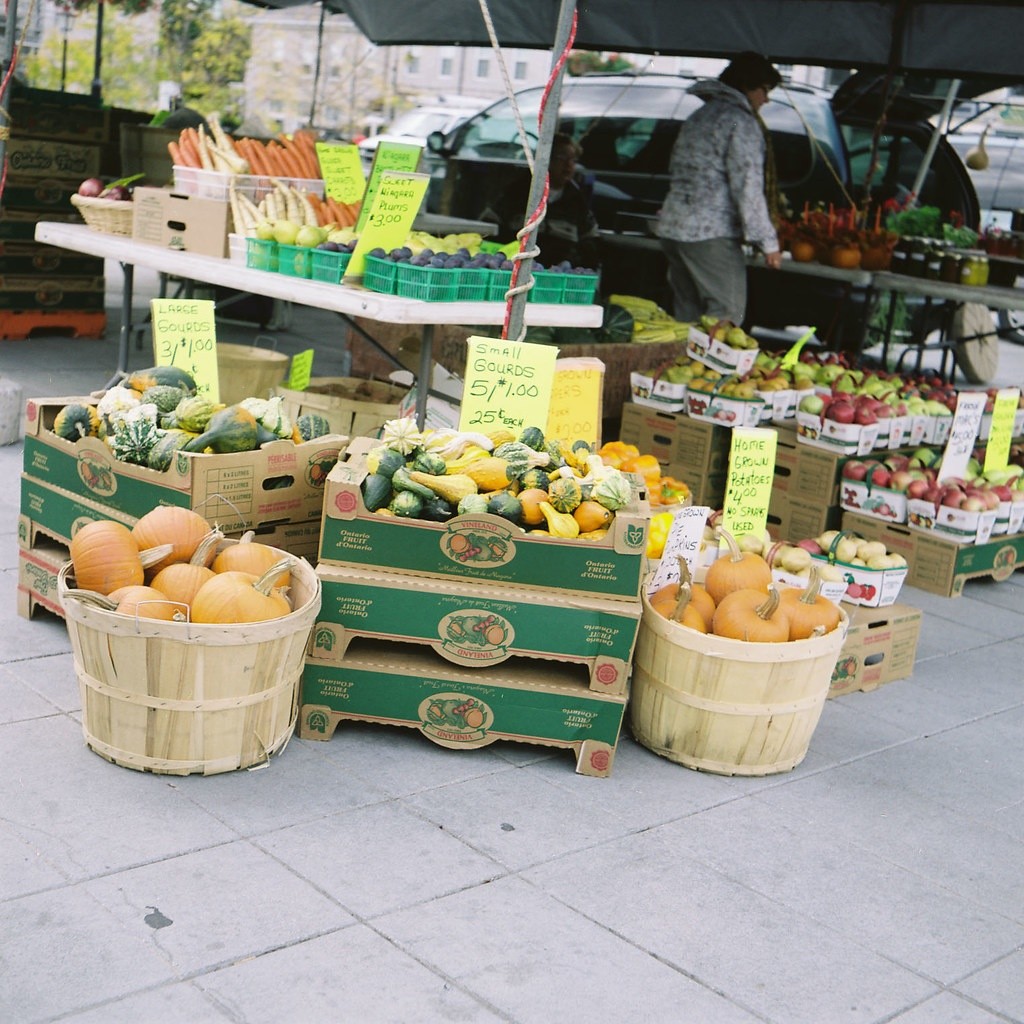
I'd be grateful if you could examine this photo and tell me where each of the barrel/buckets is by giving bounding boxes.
[58,538,321,777]
[215,340,289,401]
[280,374,410,438]
[634,566,849,775]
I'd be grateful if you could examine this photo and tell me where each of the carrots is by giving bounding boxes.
[168,128,361,228]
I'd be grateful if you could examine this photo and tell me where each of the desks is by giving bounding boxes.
[753,258,873,360]
[870,272,1024,385]
[31,222,604,434]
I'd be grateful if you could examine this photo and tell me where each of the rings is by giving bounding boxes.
[773,259,778,263]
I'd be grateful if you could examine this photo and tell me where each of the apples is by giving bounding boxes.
[635,321,1024,511]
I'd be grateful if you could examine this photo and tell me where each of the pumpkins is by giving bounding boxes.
[54,366,840,644]
[598,294,703,345]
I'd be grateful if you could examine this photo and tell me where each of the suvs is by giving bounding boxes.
[424,72,982,351]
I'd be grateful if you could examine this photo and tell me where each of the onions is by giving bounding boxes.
[78,178,103,195]
[111,186,129,200]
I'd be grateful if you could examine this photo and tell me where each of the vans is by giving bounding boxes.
[355,101,536,170]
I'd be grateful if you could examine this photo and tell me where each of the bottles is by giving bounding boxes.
[890,237,990,286]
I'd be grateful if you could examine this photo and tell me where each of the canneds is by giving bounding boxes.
[892,236,989,286]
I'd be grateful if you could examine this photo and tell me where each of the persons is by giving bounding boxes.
[653,48,782,330]
[477,132,605,269]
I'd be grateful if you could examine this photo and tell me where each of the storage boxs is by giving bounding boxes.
[294,644,633,779]
[766,426,945,508]
[0,88,599,315]
[657,462,728,510]
[760,482,844,545]
[319,435,653,604]
[616,402,730,474]
[306,564,645,697]
[840,510,1024,598]
[21,390,349,537]
[821,600,923,701]
[16,475,320,570]
[16,547,71,625]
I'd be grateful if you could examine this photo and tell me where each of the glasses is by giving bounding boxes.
[552,154,579,165]
[759,85,771,96]
